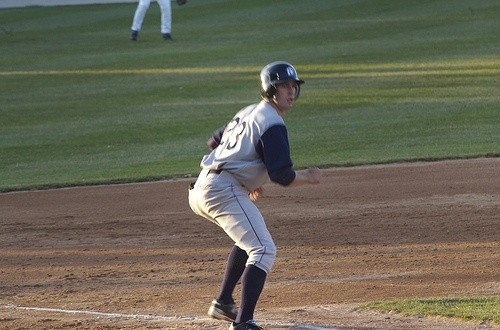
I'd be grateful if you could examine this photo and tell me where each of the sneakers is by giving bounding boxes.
[162,33,174,41]
[260,60,305,93]
[229,319,264,330]
[208,299,239,322]
[129,30,137,39]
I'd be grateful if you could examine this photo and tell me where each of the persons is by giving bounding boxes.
[128,0,175,42]
[187,61,322,330]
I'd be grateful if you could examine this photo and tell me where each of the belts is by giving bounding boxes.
[208,169,221,175]
